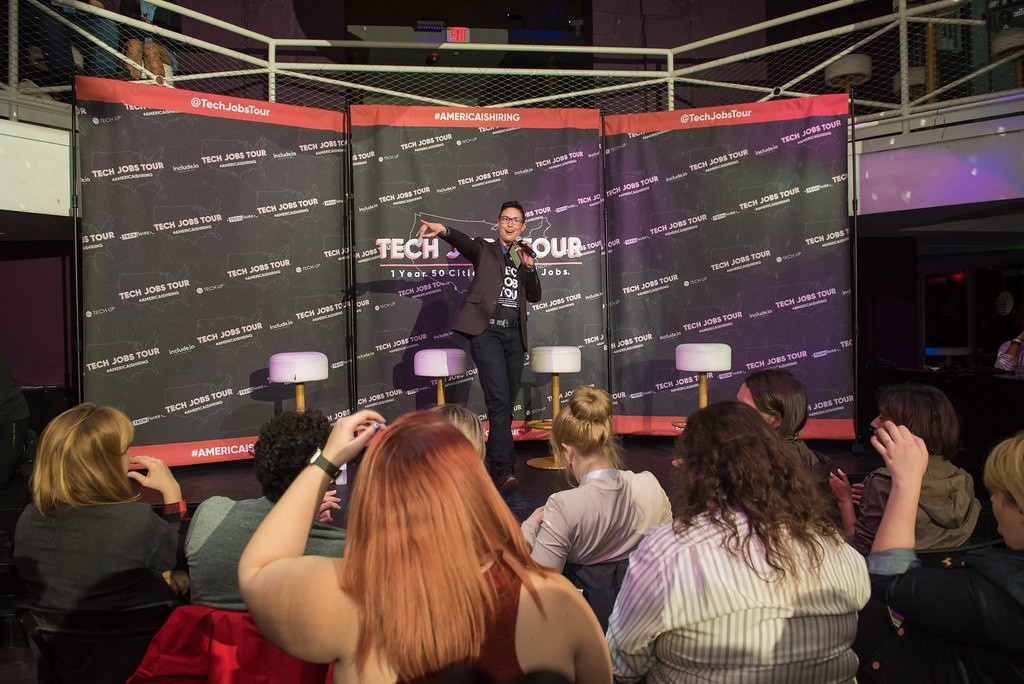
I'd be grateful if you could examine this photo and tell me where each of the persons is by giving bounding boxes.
[12,400,192,684]
[431,385,676,575]
[183,409,349,612]
[416,201,542,500]
[0,0,183,103]
[995,330,1024,375]
[605,399,872,684]
[736,368,1024,684]
[238,408,613,684]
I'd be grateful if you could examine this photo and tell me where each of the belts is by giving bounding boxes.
[489,318,520,328]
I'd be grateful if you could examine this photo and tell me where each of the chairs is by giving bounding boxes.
[15,601,176,684]
[912,537,1007,570]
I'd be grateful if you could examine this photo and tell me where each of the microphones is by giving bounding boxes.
[515,236,536,259]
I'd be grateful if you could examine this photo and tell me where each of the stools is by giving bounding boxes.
[414,348,467,406]
[672,344,732,467]
[991,28,1024,88]
[269,351,328,415]
[893,66,939,106]
[526,345,581,470]
[825,53,872,115]
[29,45,174,103]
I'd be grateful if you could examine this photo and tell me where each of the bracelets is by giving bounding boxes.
[1011,338,1022,346]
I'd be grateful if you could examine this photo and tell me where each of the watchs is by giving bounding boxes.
[305,447,343,482]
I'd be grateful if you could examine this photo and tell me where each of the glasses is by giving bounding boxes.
[499,215,524,226]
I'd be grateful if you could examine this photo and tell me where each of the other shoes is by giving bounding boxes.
[501,475,517,490]
